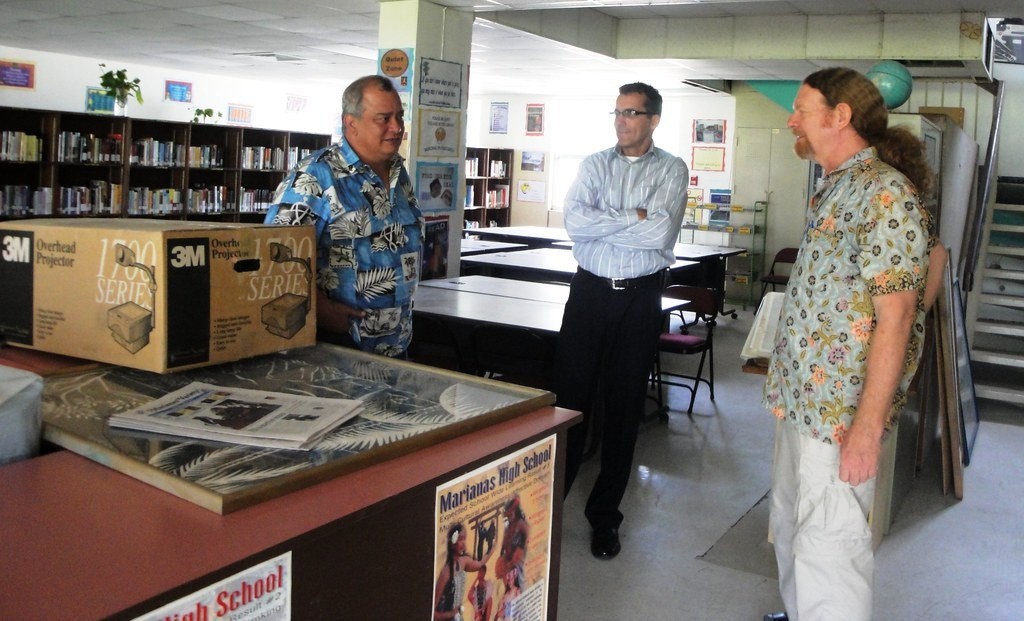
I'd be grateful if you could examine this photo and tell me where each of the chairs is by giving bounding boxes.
[644,281,725,415]
[756,249,802,313]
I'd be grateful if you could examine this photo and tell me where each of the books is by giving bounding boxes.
[0,131,309,216]
[463,157,510,239]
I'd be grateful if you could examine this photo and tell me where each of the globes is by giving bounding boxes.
[865,61,912,109]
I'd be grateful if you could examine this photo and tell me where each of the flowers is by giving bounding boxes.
[97,63,144,110]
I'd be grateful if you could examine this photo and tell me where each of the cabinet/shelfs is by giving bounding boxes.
[462,147,514,242]
[677,202,768,300]
[0,105,331,225]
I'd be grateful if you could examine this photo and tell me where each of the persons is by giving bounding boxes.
[264,75,426,360]
[761,66,948,621]
[433,498,530,621]
[548,81,689,558]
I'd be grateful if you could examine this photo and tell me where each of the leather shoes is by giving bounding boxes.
[591,525,621,560]
[763,611,789,621]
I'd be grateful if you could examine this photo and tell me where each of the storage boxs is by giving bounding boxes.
[0,217,319,374]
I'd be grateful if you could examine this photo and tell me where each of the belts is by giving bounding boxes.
[577,265,664,290]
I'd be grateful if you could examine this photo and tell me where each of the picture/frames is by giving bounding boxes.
[933,246,980,498]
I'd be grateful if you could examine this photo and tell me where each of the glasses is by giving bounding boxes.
[614,108,655,118]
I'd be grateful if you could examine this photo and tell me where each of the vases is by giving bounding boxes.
[117,96,128,116]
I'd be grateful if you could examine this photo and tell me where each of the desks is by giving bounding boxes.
[0,225,750,621]
